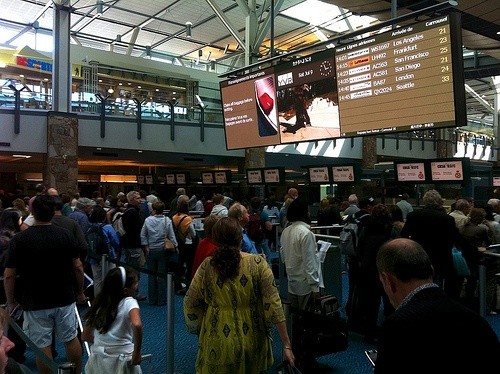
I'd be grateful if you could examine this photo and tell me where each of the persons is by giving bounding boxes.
[346,204,407,338]
[182,217,295,374]
[253,188,325,310]
[400,190,486,311]
[375,237,500,374]
[141,187,258,306]
[396,193,413,223]
[0,182,144,374]
[318,193,360,273]
[447,196,500,315]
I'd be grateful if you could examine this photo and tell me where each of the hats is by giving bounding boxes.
[47,188,58,196]
[108,195,112,198]
[363,196,377,205]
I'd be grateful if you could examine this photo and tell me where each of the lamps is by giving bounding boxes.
[199,50,202,57]
[96,0,103,17]
[34,21,39,29]
[210,60,216,71]
[145,44,151,57]
[186,24,192,37]
[117,34,121,42]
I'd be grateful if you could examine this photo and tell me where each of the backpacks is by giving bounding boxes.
[112,207,135,236]
[246,210,265,241]
[85,223,111,265]
[340,214,371,255]
[174,216,189,253]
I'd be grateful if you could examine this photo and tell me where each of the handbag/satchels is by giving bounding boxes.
[320,294,348,328]
[164,238,175,251]
[311,328,349,354]
[167,253,183,272]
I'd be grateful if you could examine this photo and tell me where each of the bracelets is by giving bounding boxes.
[284,347,292,350]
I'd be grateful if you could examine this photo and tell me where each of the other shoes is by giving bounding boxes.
[178,290,185,294]
[298,360,333,374]
[136,295,146,300]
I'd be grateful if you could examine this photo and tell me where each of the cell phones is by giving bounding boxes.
[365,349,377,367]
